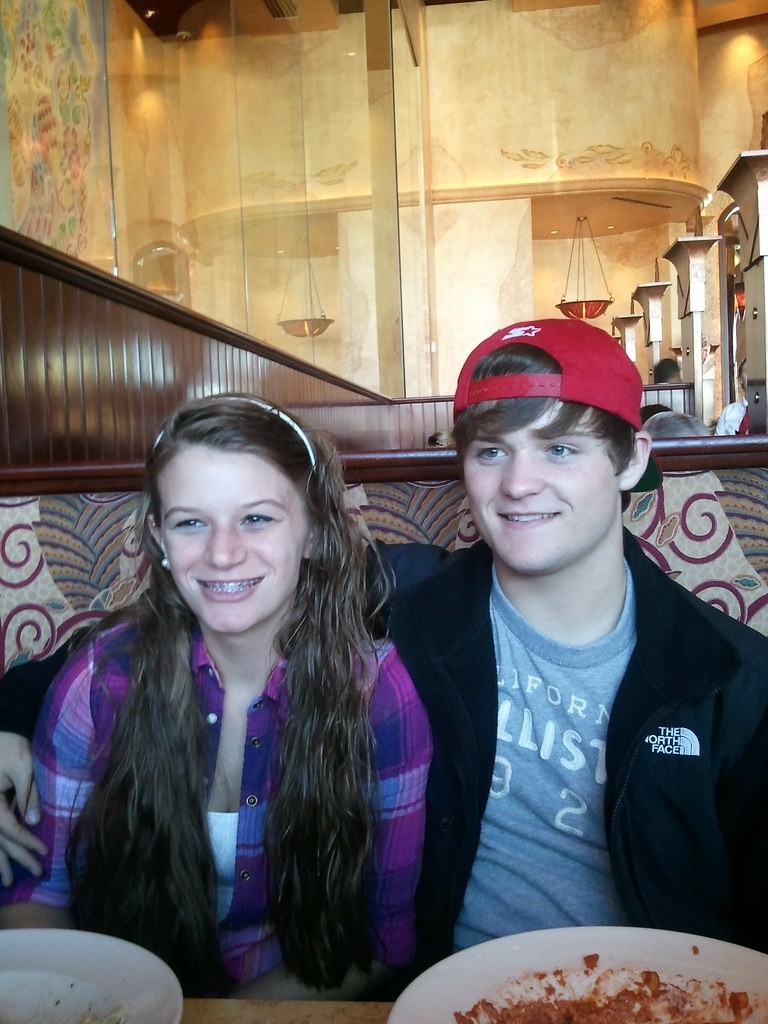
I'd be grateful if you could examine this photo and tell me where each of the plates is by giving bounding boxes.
[0,926,183,1024]
[386,924,768,1023]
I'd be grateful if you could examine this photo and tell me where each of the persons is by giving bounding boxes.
[2,320,768,1000]
[425,356,752,459]
[3,391,436,1000]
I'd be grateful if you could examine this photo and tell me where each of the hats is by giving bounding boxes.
[452,318,663,492]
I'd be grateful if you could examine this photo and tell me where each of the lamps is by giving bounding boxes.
[274,238,336,339]
[554,214,616,320]
